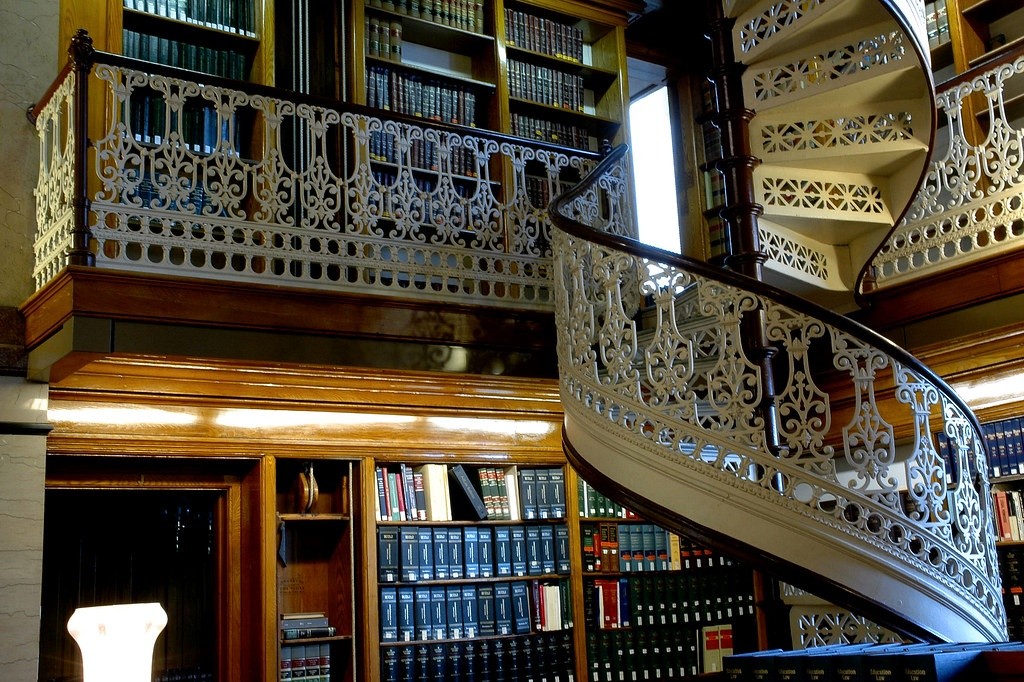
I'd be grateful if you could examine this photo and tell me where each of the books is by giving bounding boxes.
[924,0,949,48]
[276,422,1024,681]
[122,1,616,294]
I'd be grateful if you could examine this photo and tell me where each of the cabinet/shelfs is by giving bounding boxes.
[351,0,638,298]
[58,0,276,277]
[921,401,1024,675]
[800,0,1024,247]
[238,461,766,682]
